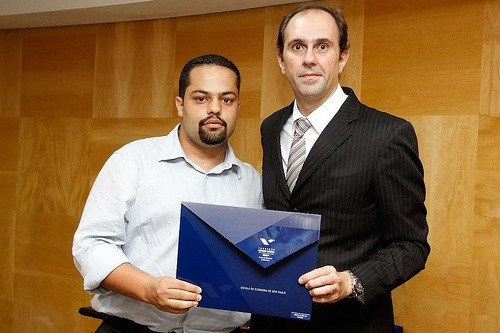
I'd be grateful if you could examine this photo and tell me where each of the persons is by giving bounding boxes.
[249,4,432,333]
[71,54,265,333]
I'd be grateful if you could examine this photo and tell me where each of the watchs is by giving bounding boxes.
[344,270,365,299]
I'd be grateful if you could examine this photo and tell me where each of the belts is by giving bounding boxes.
[79,307,176,333]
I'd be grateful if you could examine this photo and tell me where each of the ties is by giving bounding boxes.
[286,117,312,194]
